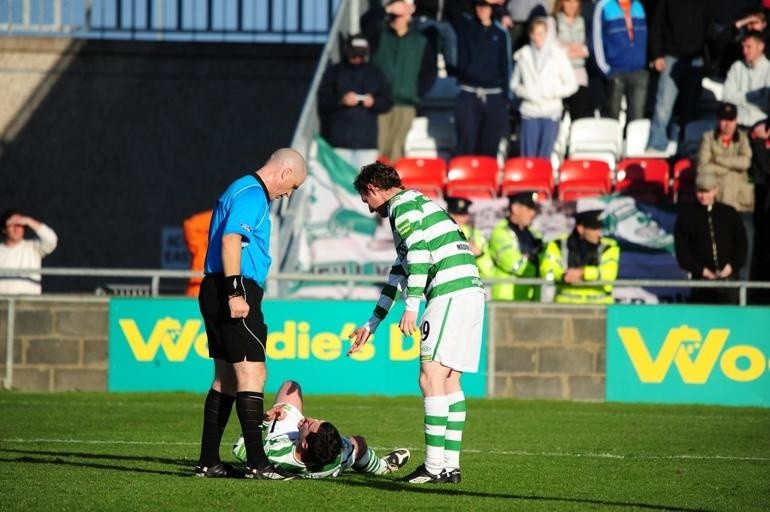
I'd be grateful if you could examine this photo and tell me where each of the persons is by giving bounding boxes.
[233,381,409,479]
[0,213,58,297]
[344,162,487,484]
[196,146,306,482]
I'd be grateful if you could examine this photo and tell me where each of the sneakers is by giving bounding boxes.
[382,448,460,483]
[195,463,241,478]
[243,462,296,481]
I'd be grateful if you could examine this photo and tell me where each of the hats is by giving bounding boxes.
[696,173,718,190]
[508,191,539,207]
[444,197,471,213]
[349,35,368,56]
[719,104,736,119]
[572,210,603,228]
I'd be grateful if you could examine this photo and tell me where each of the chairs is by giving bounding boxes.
[304,76,770,199]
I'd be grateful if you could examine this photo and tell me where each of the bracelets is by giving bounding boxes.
[223,273,245,298]
